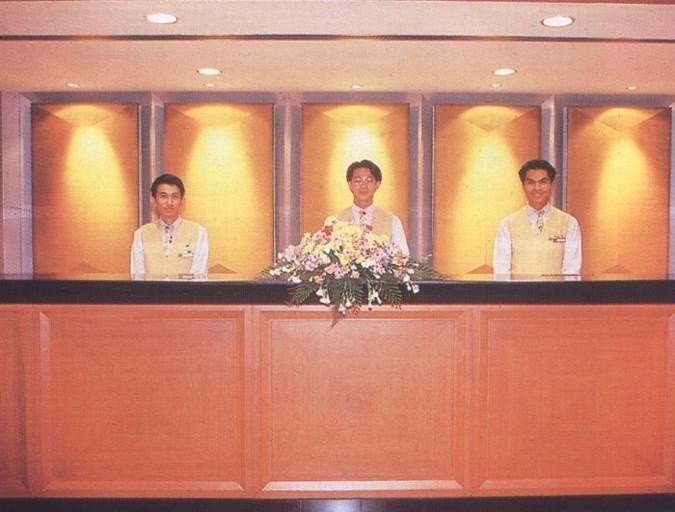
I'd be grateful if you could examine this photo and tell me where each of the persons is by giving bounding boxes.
[491,159,585,275]
[329,159,410,258]
[126,173,208,274]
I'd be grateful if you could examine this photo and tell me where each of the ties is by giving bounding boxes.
[163,226,171,258]
[358,210,366,222]
[536,209,545,233]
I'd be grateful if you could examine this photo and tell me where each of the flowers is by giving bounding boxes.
[255,216,453,335]
